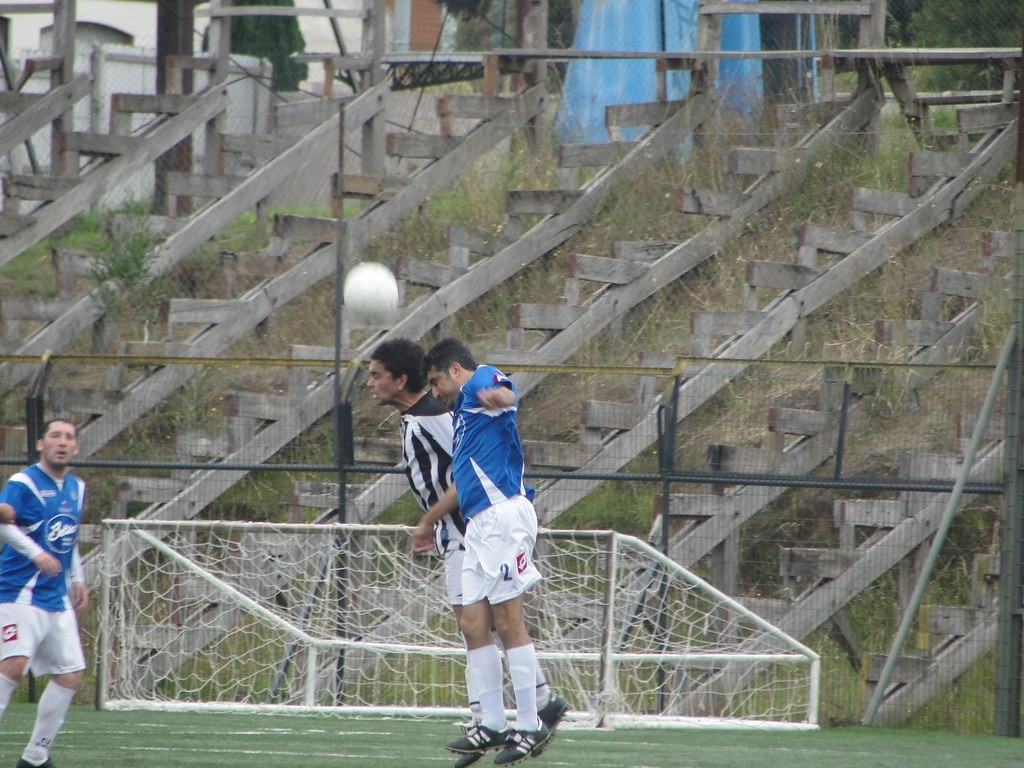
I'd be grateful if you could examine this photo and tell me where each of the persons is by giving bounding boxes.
[0,418,91,768]
[367,338,570,768]
[411,338,554,766]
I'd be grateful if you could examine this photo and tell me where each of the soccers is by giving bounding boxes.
[343,265,399,327]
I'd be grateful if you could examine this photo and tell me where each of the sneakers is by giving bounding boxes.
[446,721,511,755]
[532,693,569,757]
[494,714,550,767]
[454,718,489,768]
[16,755,56,768]
